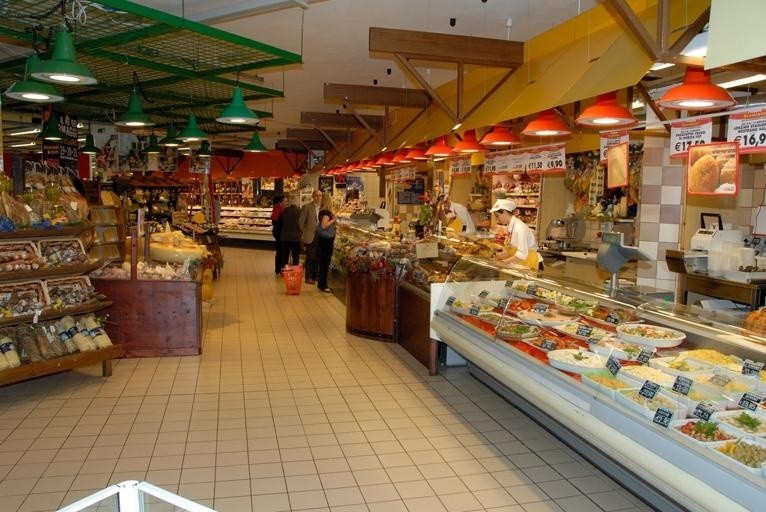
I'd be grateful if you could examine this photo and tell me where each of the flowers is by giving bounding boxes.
[340,255,395,273]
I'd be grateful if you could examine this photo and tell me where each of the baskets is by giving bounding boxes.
[283,264,304,294]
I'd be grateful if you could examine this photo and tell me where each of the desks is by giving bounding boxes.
[676,271,765,312]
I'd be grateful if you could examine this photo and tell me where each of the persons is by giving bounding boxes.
[489,199,544,277]
[317,194,340,293]
[285,197,301,265]
[298,190,322,284]
[439,195,476,238]
[271,196,289,274]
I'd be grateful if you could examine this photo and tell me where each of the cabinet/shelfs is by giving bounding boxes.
[327,217,534,371]
[111,171,208,260]
[1,211,124,385]
[429,279,765,511]
[211,206,278,248]
[491,172,543,229]
[81,179,212,358]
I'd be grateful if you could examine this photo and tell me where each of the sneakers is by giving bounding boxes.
[324,287,333,292]
[305,278,314,284]
[274,273,281,278]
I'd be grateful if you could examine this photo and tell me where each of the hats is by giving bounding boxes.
[489,199,516,212]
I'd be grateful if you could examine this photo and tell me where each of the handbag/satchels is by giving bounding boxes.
[318,222,336,238]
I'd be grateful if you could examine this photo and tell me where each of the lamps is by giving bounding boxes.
[352,165,363,171]
[365,160,382,167]
[1,26,67,103]
[216,68,260,124]
[405,146,428,160]
[391,149,415,163]
[36,103,62,140]
[339,166,346,172]
[452,129,488,152]
[333,169,341,174]
[346,164,353,172]
[157,114,184,146]
[376,153,396,165]
[114,72,155,126]
[355,161,371,168]
[142,134,162,154]
[479,125,521,145]
[78,106,100,154]
[575,92,638,129]
[654,67,737,112]
[195,140,214,157]
[29,1,99,86]
[175,92,209,142]
[325,169,335,176]
[425,136,457,156]
[521,109,571,136]
[242,124,269,152]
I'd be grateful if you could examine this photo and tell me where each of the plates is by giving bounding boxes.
[615,388,689,421]
[449,302,495,316]
[708,240,766,278]
[710,410,766,437]
[679,351,744,368]
[475,312,521,326]
[581,373,644,400]
[616,324,686,348]
[668,418,740,450]
[589,338,657,362]
[480,294,521,308]
[494,324,542,341]
[516,309,582,327]
[687,373,755,409]
[648,356,715,377]
[502,303,517,316]
[530,295,555,305]
[521,337,588,353]
[552,322,617,342]
[706,439,766,483]
[658,386,729,418]
[578,312,645,327]
[547,349,608,374]
[618,365,677,385]
[555,302,596,315]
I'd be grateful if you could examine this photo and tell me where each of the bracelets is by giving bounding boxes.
[330,219,336,223]
